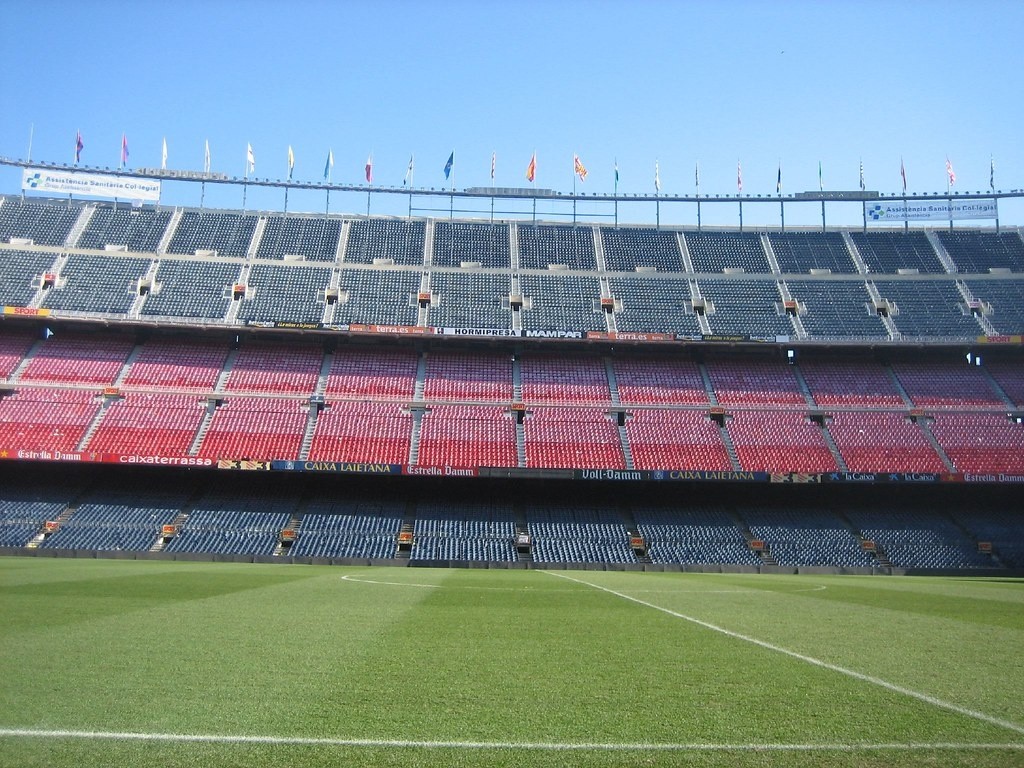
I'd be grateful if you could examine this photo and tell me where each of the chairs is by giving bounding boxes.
[0,202,1024,572]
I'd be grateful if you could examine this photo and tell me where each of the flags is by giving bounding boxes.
[901,162,906,189]
[575,156,587,182]
[527,156,535,181]
[324,153,333,180]
[365,157,371,182]
[947,159,954,185]
[404,160,412,185]
[655,167,660,190]
[990,160,994,188]
[738,162,742,191]
[206,143,211,171]
[444,152,453,179]
[248,145,255,172]
[860,162,865,189]
[122,136,129,167]
[163,142,167,169]
[288,148,294,178]
[76,133,84,163]
[777,171,781,192]
